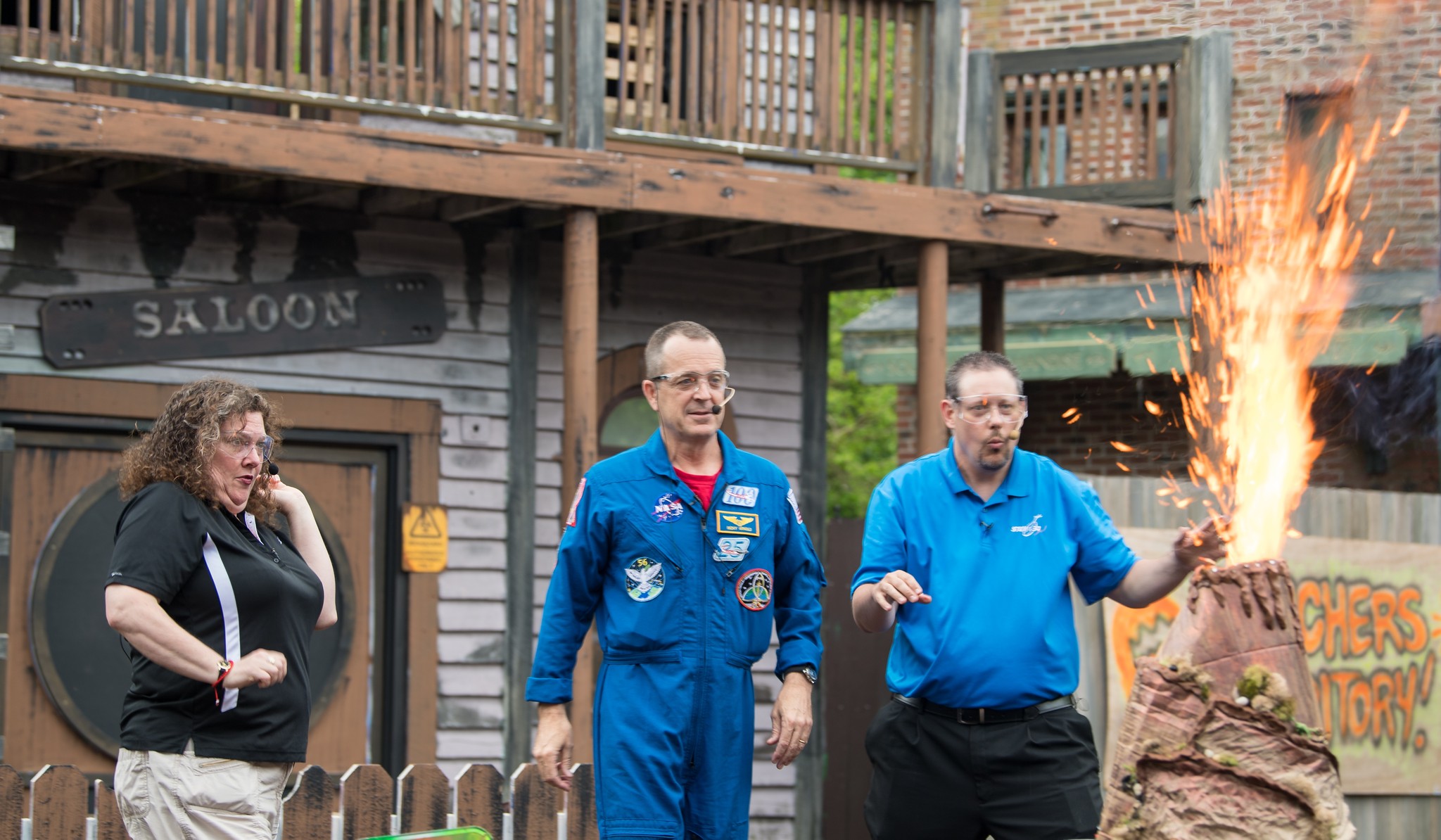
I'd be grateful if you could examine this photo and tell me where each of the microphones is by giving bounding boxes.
[712,387,735,415]
[1009,411,1026,440]
[263,452,279,475]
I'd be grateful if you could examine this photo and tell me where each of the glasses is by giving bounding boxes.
[952,394,1028,423]
[212,431,274,463]
[651,369,730,392]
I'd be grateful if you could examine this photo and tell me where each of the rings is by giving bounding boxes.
[268,657,275,665]
[799,739,806,743]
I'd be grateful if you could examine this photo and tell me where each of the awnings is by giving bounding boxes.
[1131,310,1421,375]
[857,320,1127,385]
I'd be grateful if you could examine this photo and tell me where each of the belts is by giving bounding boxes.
[893,691,1072,724]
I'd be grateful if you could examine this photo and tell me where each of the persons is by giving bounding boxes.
[105,379,337,840]
[525,320,827,840]
[849,352,1238,840]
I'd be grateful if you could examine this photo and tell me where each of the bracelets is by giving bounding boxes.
[212,661,234,706]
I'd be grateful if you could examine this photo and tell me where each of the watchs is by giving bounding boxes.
[782,665,818,685]
[216,659,231,692]
